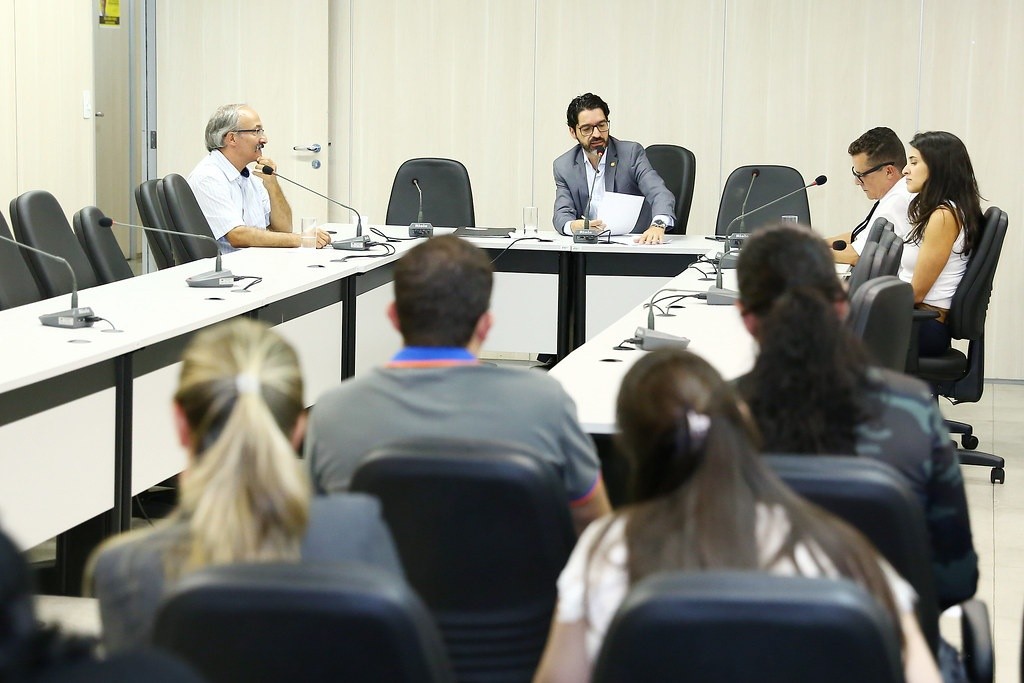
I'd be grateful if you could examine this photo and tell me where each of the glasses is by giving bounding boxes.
[224,127,264,139]
[852,160,894,183]
[577,119,610,136]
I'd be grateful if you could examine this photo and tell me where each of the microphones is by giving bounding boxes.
[262,166,372,250]
[98,218,235,287]
[633,168,846,352]
[409,178,434,237]
[574,146,605,244]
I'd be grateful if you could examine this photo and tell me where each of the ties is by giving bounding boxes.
[851,199,880,244]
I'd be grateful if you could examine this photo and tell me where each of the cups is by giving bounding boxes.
[523,207,538,237]
[301,218,317,251]
[781,216,798,225]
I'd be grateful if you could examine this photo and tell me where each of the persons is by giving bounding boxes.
[552,93,676,244]
[82,313,405,655]
[181,104,331,248]
[822,128,986,360]
[532,346,944,683]
[300,233,613,543]
[0,533,200,683]
[707,224,978,618]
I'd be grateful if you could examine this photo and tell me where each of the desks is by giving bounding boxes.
[0,221,853,644]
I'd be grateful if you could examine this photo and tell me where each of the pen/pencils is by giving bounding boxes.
[326,231,337,234]
[581,215,603,231]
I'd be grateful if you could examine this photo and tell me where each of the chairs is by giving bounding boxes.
[385,157,476,227]
[147,558,454,683]
[0,172,223,312]
[595,143,1011,683]
[349,435,576,682]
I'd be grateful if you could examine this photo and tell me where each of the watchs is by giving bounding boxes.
[651,220,666,229]
